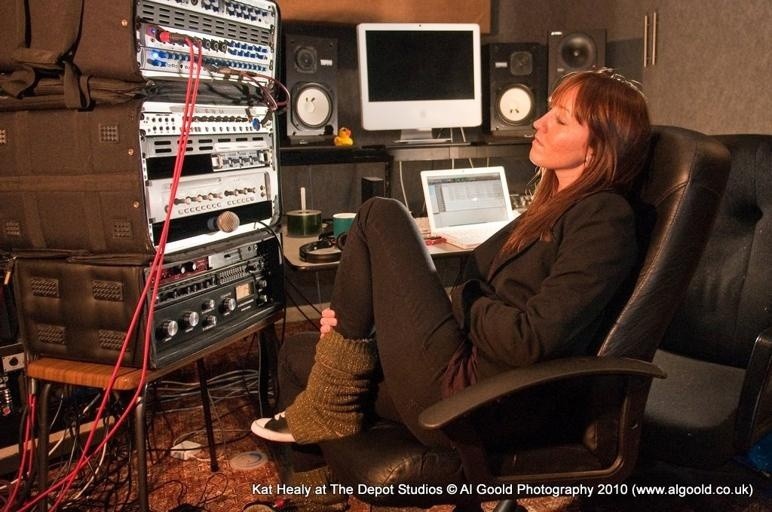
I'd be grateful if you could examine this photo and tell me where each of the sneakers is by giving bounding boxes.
[249,408,292,443]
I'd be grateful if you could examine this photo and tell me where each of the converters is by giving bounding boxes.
[170,440,202,461]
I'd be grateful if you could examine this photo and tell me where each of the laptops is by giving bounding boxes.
[420,165,513,249]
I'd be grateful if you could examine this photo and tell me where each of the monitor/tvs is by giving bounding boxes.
[356,21,481,142]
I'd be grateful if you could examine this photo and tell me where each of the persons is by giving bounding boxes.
[249,65,653,511]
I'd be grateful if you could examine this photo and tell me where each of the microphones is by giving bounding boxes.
[204,211,239,233]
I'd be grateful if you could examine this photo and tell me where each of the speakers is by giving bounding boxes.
[487,40,545,136]
[281,30,339,146]
[361,176,384,203]
[548,26,605,98]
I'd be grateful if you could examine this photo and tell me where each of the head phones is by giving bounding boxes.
[298,230,347,262]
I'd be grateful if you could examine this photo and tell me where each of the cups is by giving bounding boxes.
[332,211,359,241]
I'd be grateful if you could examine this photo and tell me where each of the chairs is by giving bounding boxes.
[267,122,732,512]
[642,131,770,465]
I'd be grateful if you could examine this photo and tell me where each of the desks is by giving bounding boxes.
[273,133,533,476]
[24,306,290,511]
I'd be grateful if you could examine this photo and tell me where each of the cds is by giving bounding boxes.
[286,208,322,237]
